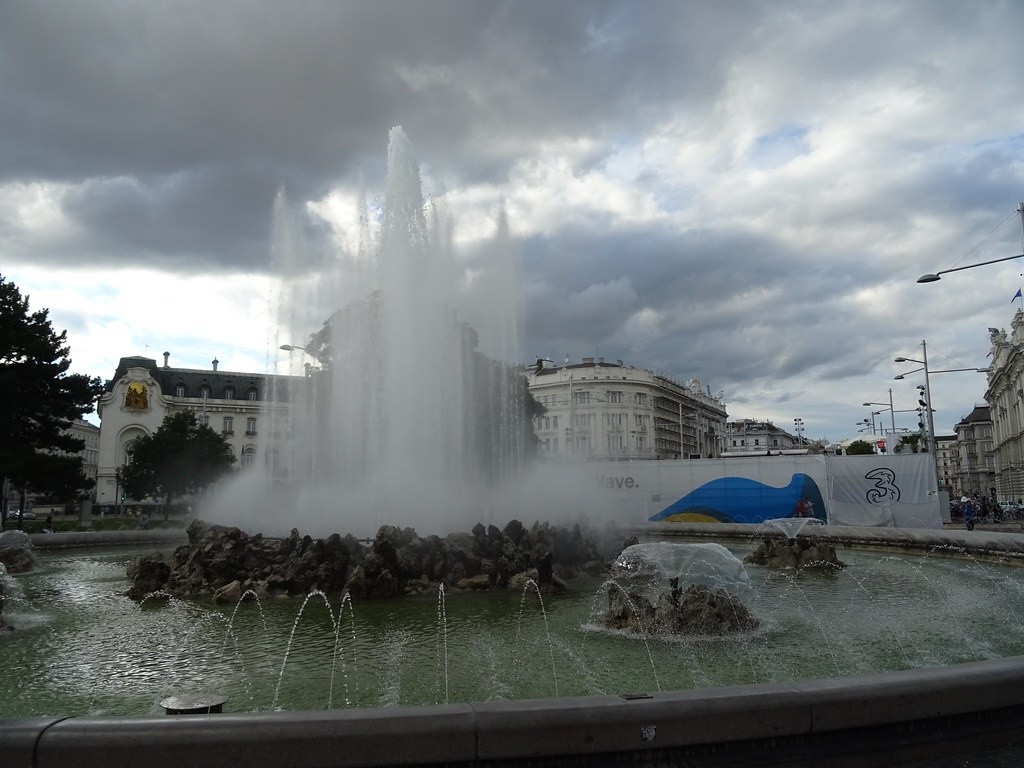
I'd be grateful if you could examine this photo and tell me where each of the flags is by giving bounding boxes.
[1010,288,1021,304]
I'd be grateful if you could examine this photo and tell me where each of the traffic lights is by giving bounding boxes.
[120,495,125,502]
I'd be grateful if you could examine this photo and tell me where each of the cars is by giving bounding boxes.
[949,498,1024,521]
[13,508,37,520]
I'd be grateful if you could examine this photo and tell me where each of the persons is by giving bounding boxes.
[962,492,1003,530]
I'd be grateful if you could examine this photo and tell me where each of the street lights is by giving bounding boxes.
[863,387,936,435]
[893,339,992,474]
[855,411,909,435]
